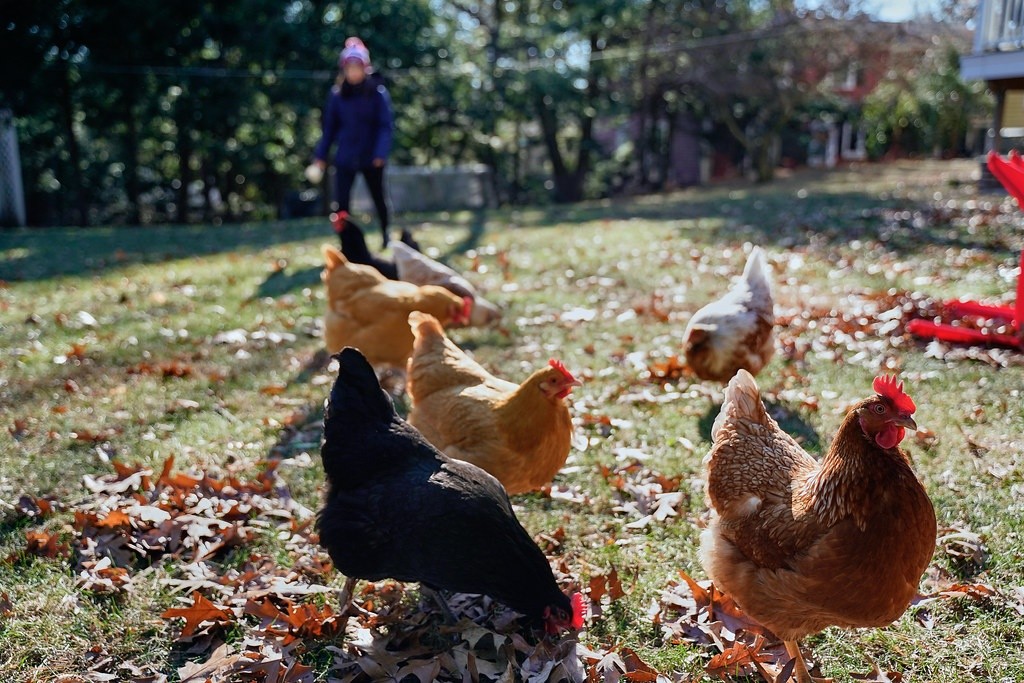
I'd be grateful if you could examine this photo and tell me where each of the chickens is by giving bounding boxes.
[404,309,582,499]
[314,346,588,643]
[319,210,504,381]
[696,369,937,683]
[686,245,774,385]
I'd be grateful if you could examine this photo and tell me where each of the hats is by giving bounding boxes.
[339,37,370,74]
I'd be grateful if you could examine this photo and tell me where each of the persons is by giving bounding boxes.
[311,45,394,254]
